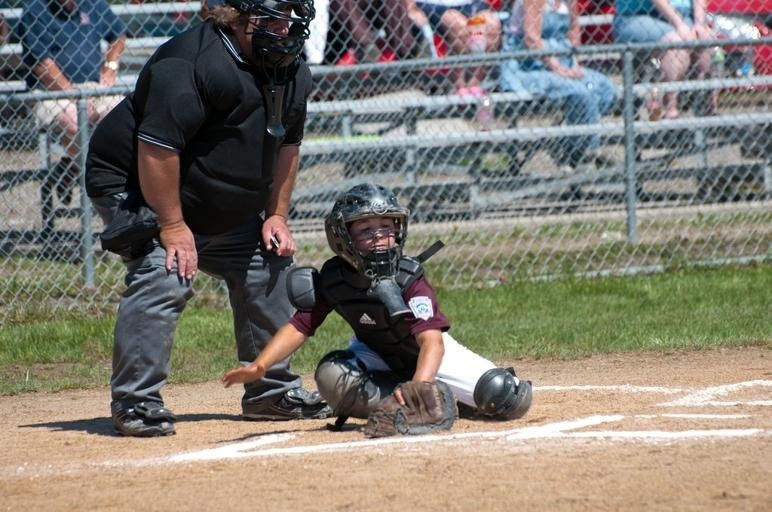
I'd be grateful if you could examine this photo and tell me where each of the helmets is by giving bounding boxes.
[323,182,412,279]
[222,0,316,86]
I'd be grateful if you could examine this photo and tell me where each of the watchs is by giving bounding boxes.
[101,60,120,72]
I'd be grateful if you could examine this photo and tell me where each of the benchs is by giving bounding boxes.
[1,2,772,232]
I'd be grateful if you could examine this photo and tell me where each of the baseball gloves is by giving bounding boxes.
[362,379,457,440]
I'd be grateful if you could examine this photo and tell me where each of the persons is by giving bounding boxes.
[80,0,335,440]
[18,0,130,208]
[313,1,772,202]
[216,182,538,430]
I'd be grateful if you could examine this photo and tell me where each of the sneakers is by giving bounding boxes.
[48,156,79,205]
[239,383,335,422]
[109,397,176,438]
